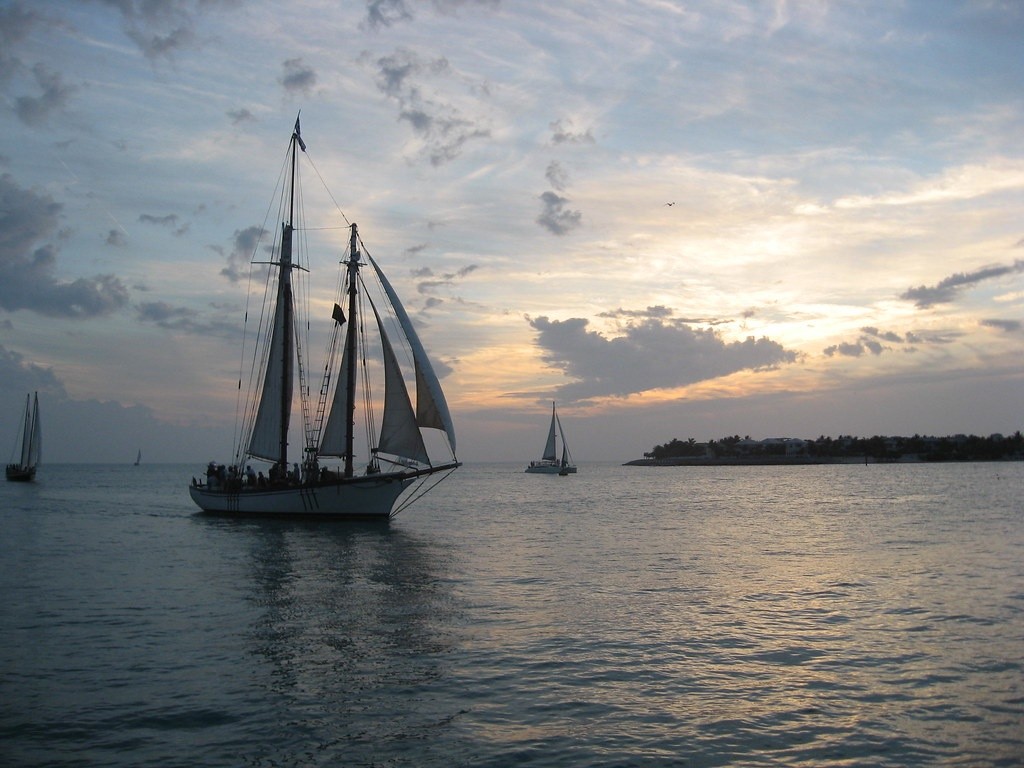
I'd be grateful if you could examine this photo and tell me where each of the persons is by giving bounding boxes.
[293,463,300,482]
[206,462,265,488]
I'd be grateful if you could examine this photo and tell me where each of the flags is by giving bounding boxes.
[332,303,346,326]
[295,115,306,152]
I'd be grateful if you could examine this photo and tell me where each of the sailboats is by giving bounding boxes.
[189,109,463,519]
[559,443,568,476]
[6,391,43,483]
[525,401,577,473]
[134,450,141,466]
